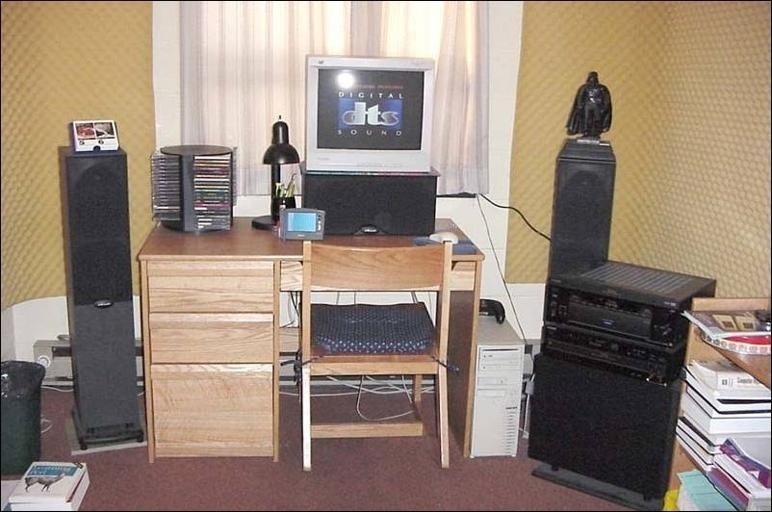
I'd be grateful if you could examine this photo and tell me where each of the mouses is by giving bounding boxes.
[429,230,460,247]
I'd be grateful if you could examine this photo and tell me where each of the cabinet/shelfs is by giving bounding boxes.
[665,296,772,511]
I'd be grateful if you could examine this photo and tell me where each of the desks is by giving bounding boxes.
[136,216,485,465]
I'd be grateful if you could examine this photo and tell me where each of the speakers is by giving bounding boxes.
[57,144,142,446]
[301,176,438,237]
[547,140,615,276]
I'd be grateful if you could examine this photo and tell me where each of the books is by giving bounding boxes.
[8,461,90,511]
[662,309,772,512]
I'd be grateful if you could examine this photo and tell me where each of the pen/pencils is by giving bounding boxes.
[274,173,297,198]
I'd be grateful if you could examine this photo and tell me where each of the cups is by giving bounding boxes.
[271,196,296,221]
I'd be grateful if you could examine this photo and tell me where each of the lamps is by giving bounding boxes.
[250,115,301,230]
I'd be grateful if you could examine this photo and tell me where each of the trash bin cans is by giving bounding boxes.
[0,360,45,475]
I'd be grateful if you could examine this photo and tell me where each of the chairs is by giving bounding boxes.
[301,240,452,474]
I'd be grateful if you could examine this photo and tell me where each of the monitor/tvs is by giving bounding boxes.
[305,55,437,173]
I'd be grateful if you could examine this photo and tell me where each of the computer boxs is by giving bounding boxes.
[470,314,526,457]
